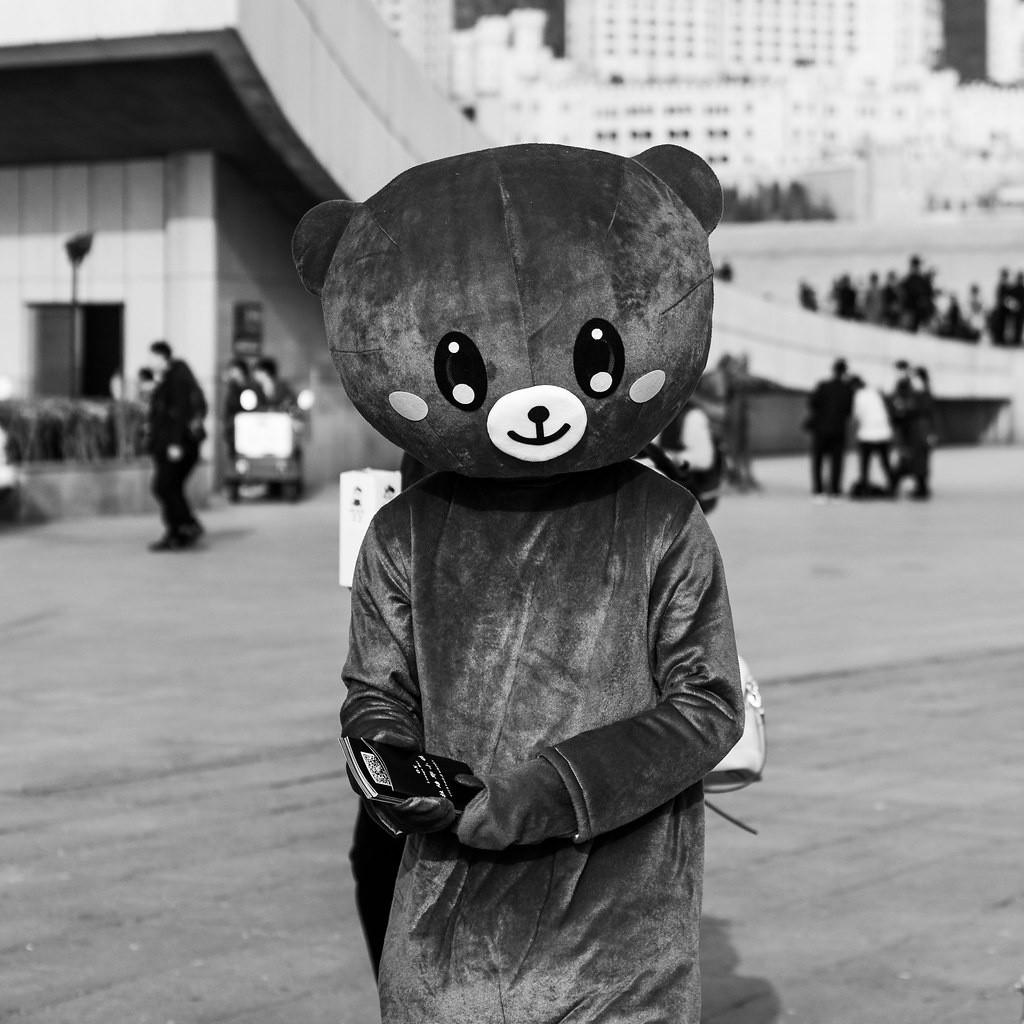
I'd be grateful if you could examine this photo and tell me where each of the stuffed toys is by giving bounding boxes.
[288,141,746,1024]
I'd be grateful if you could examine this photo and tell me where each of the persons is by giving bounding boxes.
[136,339,311,551]
[646,253,1024,513]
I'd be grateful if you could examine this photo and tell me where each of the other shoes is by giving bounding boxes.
[812,479,935,500]
[151,529,201,551]
